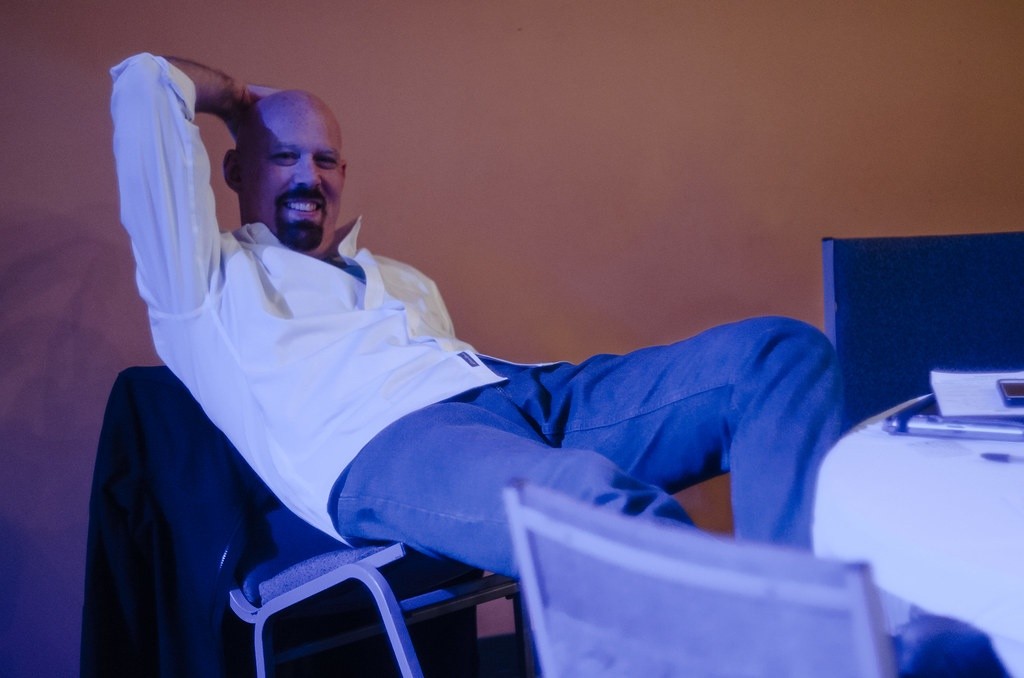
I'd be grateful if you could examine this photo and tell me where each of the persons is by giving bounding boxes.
[111,52,1014,678]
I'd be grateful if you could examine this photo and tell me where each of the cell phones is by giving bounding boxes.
[997,378,1024,408]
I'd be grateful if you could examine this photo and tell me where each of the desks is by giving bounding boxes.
[811,388,1024,678]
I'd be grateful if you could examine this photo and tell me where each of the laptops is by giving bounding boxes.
[883,393,1024,441]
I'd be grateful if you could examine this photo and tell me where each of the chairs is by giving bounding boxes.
[816,233,1024,434]
[124,363,541,678]
[500,478,902,677]
